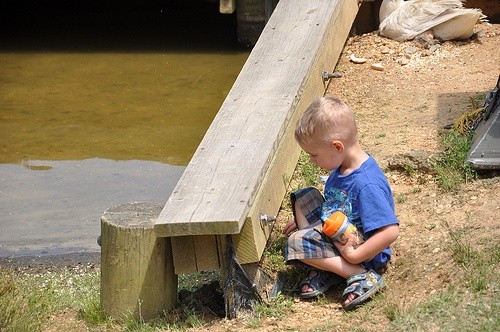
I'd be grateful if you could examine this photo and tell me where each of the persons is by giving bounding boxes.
[280,96,400,308]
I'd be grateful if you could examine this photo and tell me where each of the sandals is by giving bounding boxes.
[342,268,386,311]
[298,266,343,298]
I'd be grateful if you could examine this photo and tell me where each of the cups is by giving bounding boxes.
[322,211,374,262]
